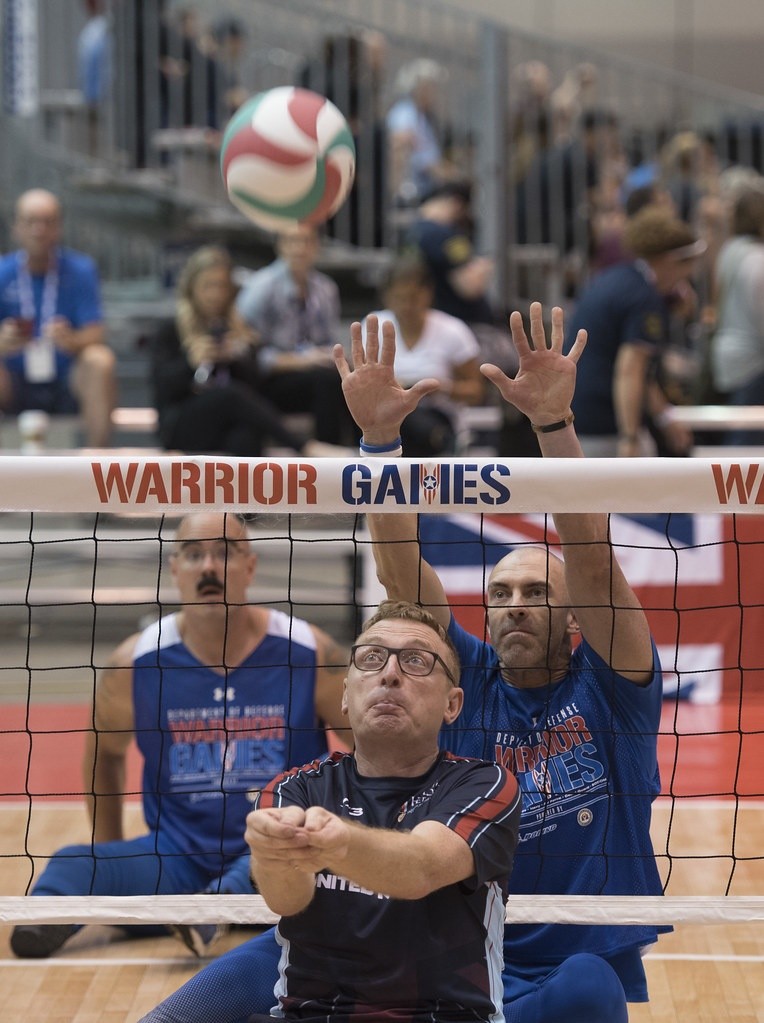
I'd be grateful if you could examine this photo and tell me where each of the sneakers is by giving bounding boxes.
[169,924,218,958]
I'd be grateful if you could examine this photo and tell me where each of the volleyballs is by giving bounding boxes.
[216,83,357,239]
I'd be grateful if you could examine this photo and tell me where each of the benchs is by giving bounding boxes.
[113,402,764,433]
[36,89,572,309]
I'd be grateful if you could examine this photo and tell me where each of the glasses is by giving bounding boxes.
[348,643,459,689]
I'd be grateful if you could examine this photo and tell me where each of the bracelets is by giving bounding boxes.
[530,411,574,433]
[358,436,403,457]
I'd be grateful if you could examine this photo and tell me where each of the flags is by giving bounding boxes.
[363,512,728,704]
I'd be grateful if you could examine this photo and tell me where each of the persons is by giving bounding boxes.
[243,598,523,1023]
[9,508,356,961]
[0,0,764,526]
[133,298,677,1023]
